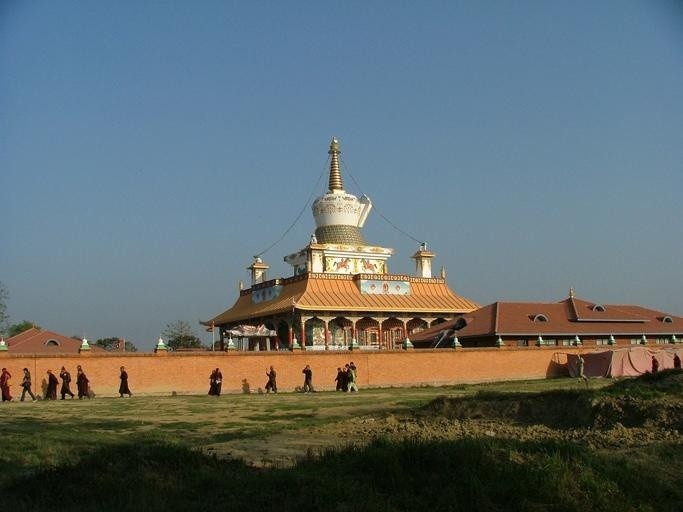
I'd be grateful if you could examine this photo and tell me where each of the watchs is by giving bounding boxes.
[301,364,313,394]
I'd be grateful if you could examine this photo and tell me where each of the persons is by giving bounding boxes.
[75,369,90,401]
[651,355,661,374]
[17,367,37,403]
[574,354,589,385]
[207,367,222,396]
[334,361,358,392]
[118,365,132,398]
[672,352,682,368]
[264,363,277,394]
[0,367,13,402]
[44,368,59,401]
[59,365,74,400]
[75,365,83,385]
